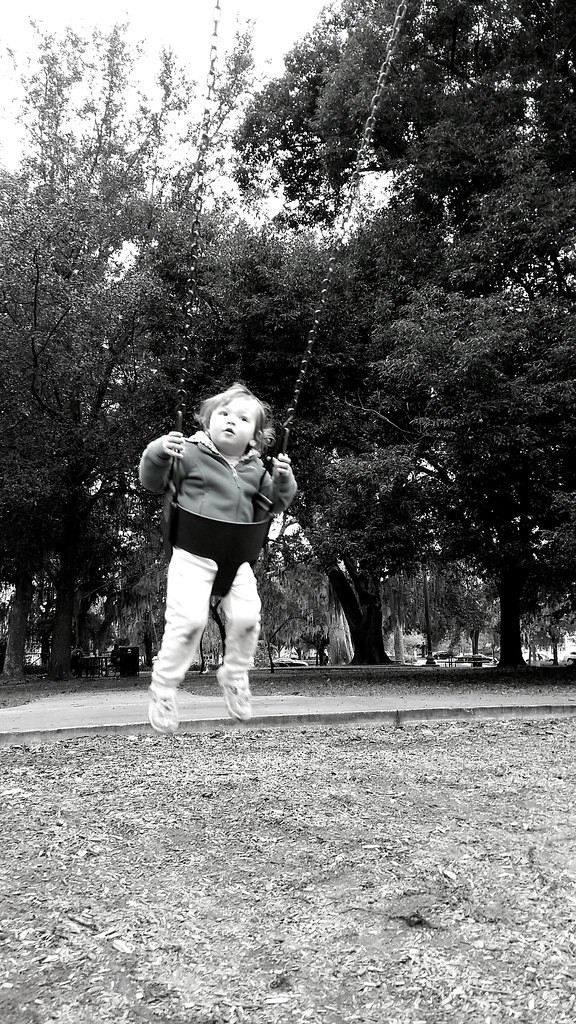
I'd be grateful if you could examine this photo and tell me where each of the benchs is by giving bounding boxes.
[80,656,119,679]
[438,653,490,669]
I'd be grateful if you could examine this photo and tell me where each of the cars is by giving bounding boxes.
[559,651,576,668]
[273,657,308,667]
[463,652,498,665]
[433,650,453,661]
[526,651,555,665]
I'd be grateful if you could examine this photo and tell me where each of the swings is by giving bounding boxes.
[159,0,412,598]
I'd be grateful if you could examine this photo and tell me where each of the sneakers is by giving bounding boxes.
[217,666,251,721]
[148,690,179,733]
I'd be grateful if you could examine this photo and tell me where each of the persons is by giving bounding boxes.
[138,382,298,734]
